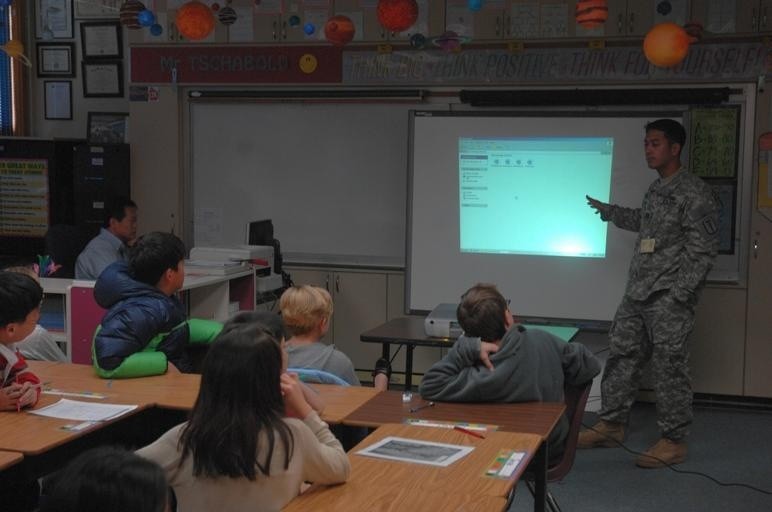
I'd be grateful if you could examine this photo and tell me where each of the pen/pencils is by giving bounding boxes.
[16,373,19,413]
[454,426,486,440]
[411,403,435,412]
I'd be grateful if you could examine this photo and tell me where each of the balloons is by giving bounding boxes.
[5,40,25,58]
[642,22,688,67]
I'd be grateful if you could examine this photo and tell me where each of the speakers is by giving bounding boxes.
[72,144,130,217]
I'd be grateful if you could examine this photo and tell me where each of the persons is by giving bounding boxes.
[217,310,325,420]
[36,444,172,512]
[3,265,70,364]
[573,118,721,468]
[0,269,43,414]
[132,323,352,512]
[89,231,225,380]
[420,282,602,469]
[72,198,137,280]
[279,284,392,392]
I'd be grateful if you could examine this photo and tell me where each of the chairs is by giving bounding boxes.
[501,380,592,512]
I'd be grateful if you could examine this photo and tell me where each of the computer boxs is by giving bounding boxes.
[256,273,283,292]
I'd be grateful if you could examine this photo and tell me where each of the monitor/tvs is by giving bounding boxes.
[247,219,273,275]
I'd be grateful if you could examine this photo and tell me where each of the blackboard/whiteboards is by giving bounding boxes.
[179,85,757,286]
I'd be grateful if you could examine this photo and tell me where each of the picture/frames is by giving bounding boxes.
[43,79,73,122]
[81,60,125,99]
[36,41,76,78]
[32,1,74,40]
[80,20,124,59]
[86,110,130,144]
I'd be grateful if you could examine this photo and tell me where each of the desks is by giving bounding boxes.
[360,317,582,391]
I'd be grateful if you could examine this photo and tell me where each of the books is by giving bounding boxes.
[182,258,249,276]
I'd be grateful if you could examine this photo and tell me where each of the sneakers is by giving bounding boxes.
[635,437,689,469]
[576,420,625,450]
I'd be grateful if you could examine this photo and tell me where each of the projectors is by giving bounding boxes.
[425,303,464,339]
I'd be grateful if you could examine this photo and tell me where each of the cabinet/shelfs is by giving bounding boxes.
[281,266,448,389]
[39,264,256,365]
